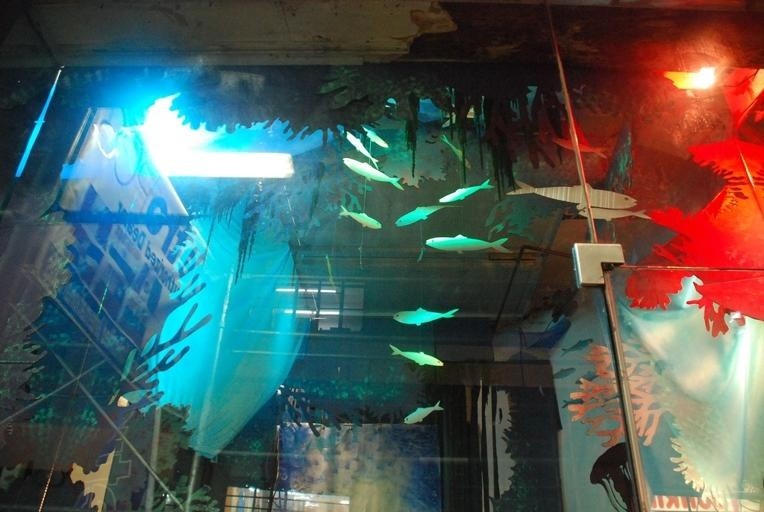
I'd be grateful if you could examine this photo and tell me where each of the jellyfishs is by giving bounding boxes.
[590,442,635,512]
